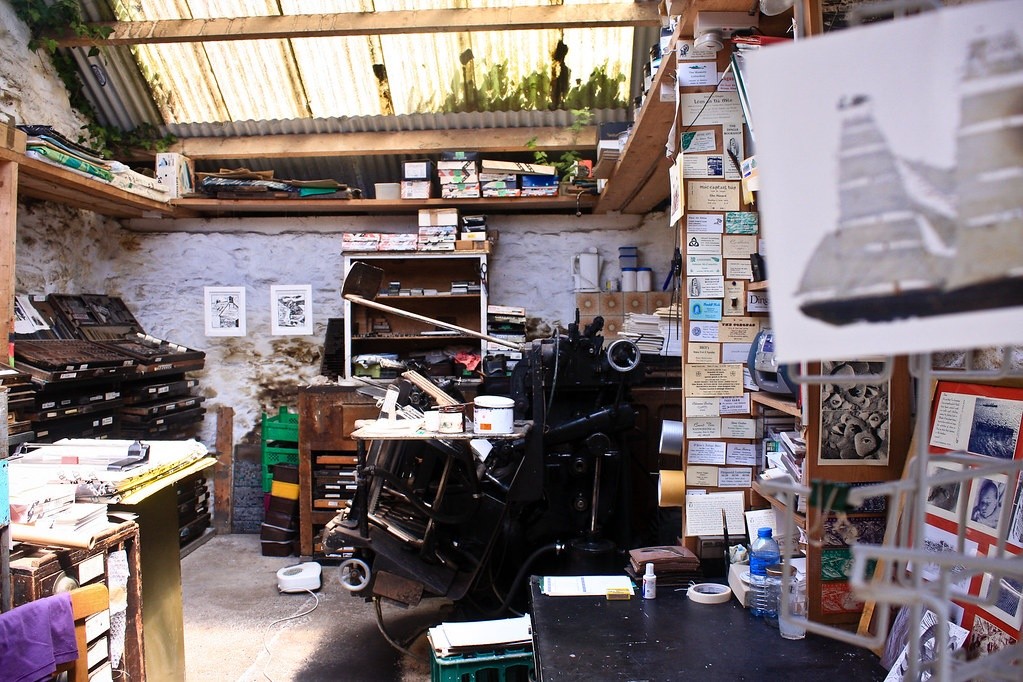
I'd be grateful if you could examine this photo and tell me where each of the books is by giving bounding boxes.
[614,303,683,356]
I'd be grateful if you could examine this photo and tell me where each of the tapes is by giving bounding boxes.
[657,470,685,508]
[660,420,684,456]
[688,583,731,604]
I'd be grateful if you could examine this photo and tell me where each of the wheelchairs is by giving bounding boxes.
[320,261,651,669]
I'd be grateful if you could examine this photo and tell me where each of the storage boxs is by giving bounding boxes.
[259,405,306,561]
[12,290,212,555]
[18,118,638,255]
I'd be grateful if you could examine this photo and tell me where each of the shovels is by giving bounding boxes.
[342,262,526,354]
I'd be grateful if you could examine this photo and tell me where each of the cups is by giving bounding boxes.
[777,594,809,640]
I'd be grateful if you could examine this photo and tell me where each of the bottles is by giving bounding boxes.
[749,527,780,617]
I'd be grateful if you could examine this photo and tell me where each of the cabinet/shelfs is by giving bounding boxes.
[294,252,686,594]
[9,510,148,682]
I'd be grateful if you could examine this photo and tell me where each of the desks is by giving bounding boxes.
[530,574,888,682]
